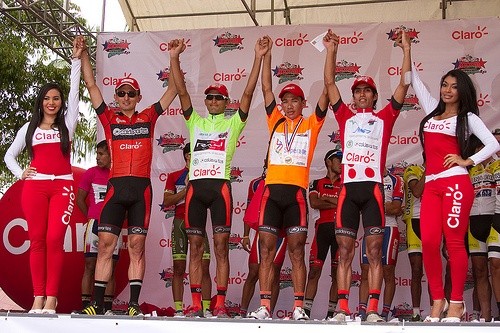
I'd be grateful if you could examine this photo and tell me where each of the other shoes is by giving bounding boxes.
[411,314,421,321]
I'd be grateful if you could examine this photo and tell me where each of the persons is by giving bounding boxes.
[443,129,500,323]
[248,29,339,319]
[77,139,121,315]
[354,168,405,321]
[169,37,269,317]
[163,143,212,316]
[303,149,343,321]
[71,34,186,316]
[404,151,434,322]
[322,30,412,322]
[231,176,287,317]
[4,38,83,313]
[395,33,500,322]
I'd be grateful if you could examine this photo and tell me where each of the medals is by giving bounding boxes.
[283,156,293,165]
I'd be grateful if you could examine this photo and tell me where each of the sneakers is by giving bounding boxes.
[78,300,103,315]
[246,305,273,320]
[328,312,347,321]
[366,314,383,322]
[185,308,202,317]
[292,306,310,321]
[213,307,230,318]
[128,301,145,317]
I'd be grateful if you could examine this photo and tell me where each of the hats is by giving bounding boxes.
[324,149,343,164]
[351,76,376,94]
[279,84,304,100]
[115,77,140,91]
[204,83,228,97]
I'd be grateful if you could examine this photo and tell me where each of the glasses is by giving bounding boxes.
[205,94,229,100]
[116,91,137,98]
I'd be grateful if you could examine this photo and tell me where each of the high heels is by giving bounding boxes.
[441,300,467,323]
[423,298,449,323]
[42,298,59,314]
[27,297,46,313]
